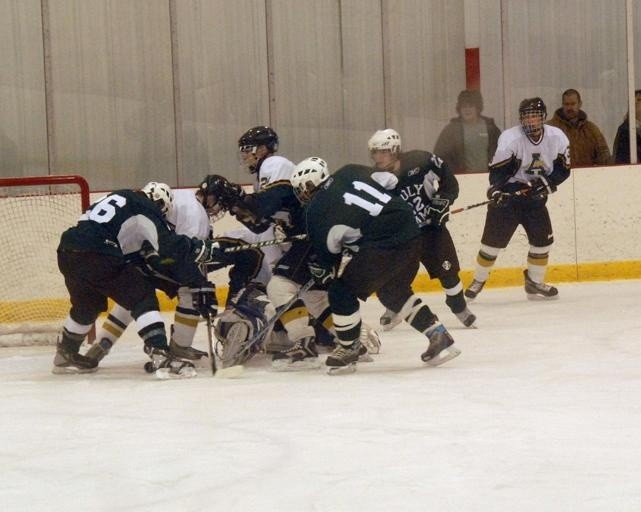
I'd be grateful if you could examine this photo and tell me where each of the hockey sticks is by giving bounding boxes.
[203,259,245,378]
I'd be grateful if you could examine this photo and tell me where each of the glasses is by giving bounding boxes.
[240,146,257,154]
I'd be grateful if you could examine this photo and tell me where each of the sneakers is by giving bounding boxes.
[381,309,401,324]
[465,279,485,299]
[421,324,453,362]
[359,323,380,354]
[324,338,366,368]
[453,308,476,326]
[524,270,558,296]
[55,346,99,373]
[270,335,320,371]
[167,341,208,361]
[266,330,295,353]
[84,344,108,365]
[314,325,335,353]
[150,349,196,379]
[221,321,251,369]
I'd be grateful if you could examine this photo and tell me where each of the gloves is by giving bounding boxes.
[527,176,550,199]
[488,186,512,209]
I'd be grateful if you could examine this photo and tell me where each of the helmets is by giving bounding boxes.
[290,156,330,207]
[238,126,278,172]
[140,182,174,217]
[195,175,234,221]
[369,129,402,155]
[519,97,547,135]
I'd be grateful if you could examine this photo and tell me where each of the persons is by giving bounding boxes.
[609,91,641,165]
[365,126,477,330]
[544,87,612,167]
[53,126,381,374]
[291,157,455,367]
[434,90,502,171]
[466,98,572,297]
